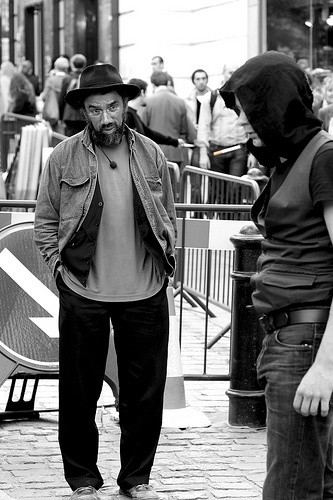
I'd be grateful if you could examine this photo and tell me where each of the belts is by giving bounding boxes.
[259,309,331,332]
[211,144,245,155]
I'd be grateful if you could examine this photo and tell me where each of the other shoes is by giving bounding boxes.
[127,484,161,500]
[70,486,101,500]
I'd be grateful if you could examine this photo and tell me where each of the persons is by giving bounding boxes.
[0,55,269,221]
[33,63,177,500]
[295,55,333,132]
[218,51,333,500]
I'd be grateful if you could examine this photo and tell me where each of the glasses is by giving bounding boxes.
[88,105,123,118]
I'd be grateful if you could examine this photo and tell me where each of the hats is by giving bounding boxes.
[64,63,142,111]
